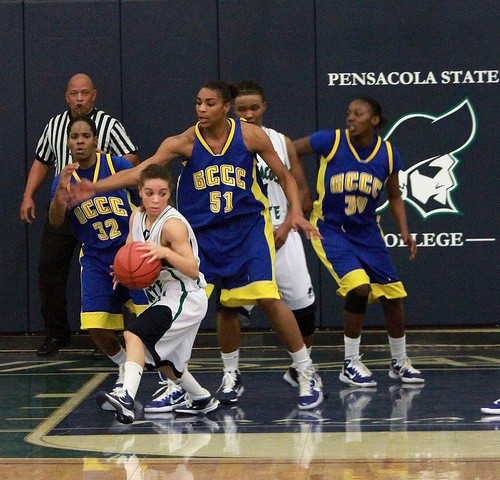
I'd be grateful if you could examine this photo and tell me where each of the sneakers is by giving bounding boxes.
[388,356,425,383]
[173,391,224,417]
[297,365,324,411]
[96,384,135,425]
[215,368,245,404]
[283,363,299,387]
[144,381,189,412]
[339,358,378,388]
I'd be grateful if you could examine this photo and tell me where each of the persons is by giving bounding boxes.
[20,74,141,358]
[292,99,424,387]
[102,164,220,424]
[47,116,142,394]
[216,78,315,406]
[60,80,324,411]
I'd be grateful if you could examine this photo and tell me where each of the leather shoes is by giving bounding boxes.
[90,348,108,359]
[37,336,71,358]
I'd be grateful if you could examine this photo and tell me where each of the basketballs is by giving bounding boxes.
[114,241,161,290]
